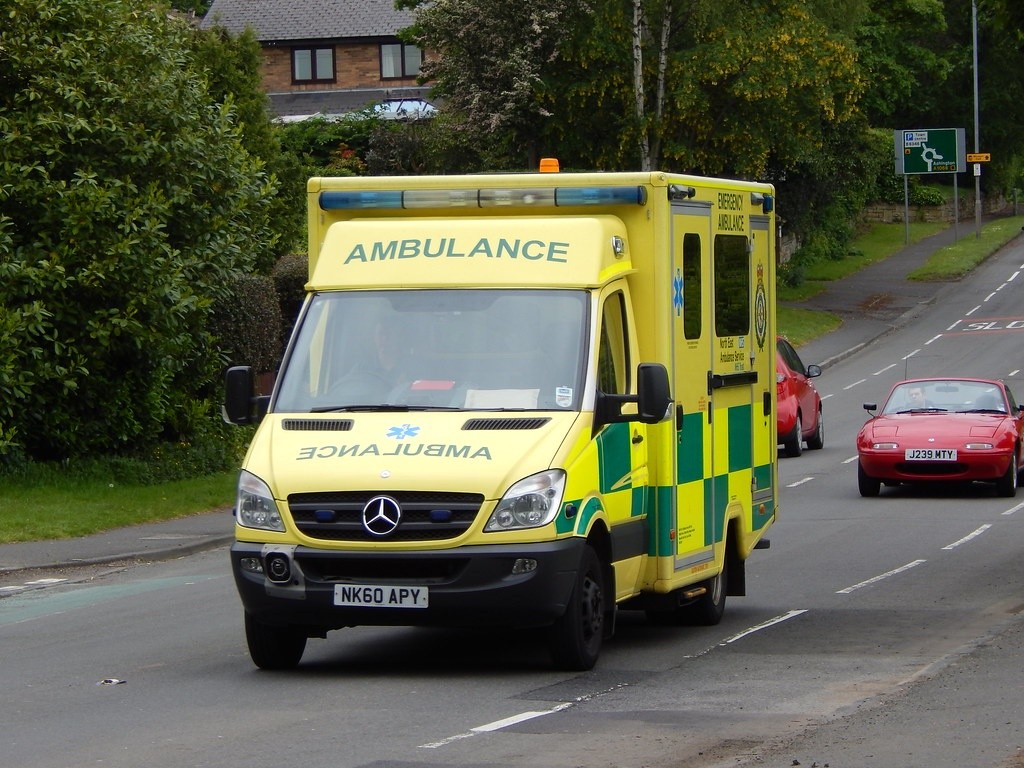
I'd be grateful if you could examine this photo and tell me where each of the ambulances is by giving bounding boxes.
[218,154,784,670]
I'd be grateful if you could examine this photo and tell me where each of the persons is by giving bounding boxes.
[904,386,927,408]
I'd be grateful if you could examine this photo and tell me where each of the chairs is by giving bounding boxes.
[974,395,998,410]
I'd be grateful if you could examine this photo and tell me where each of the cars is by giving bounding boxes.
[775,336,824,459]
[855,378,1023,497]
